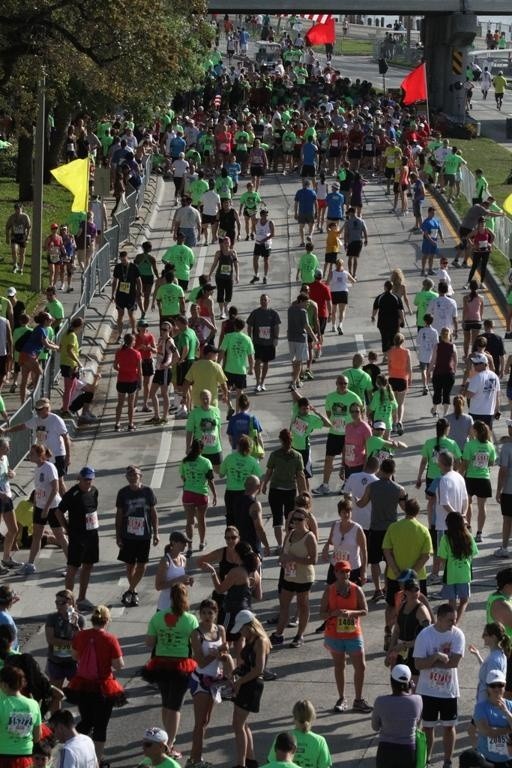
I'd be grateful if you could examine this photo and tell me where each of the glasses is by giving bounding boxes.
[143,741,153,749]
[55,599,70,607]
[224,535,239,540]
[292,516,306,522]
[487,683,504,688]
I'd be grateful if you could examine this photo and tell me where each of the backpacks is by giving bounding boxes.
[14,329,33,353]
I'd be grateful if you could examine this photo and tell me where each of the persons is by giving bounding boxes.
[2,12,512,768]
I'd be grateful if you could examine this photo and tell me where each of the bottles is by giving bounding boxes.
[67,605,75,624]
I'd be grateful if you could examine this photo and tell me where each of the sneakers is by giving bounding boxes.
[261,276,268,284]
[164,746,183,761]
[305,368,313,381]
[120,589,133,607]
[219,312,227,319]
[300,375,306,383]
[81,414,95,424]
[14,562,36,576]
[479,282,488,290]
[333,696,348,714]
[75,597,96,612]
[463,281,470,290]
[338,482,347,495]
[309,484,332,497]
[351,697,374,713]
[336,326,344,337]
[144,416,160,427]
[420,270,428,278]
[153,419,169,427]
[297,380,304,388]
[1,558,22,569]
[430,408,438,418]
[66,287,74,294]
[198,540,209,551]
[461,261,473,269]
[224,407,236,421]
[288,616,300,627]
[88,411,96,419]
[492,547,510,559]
[268,631,286,648]
[12,266,19,274]
[59,283,65,291]
[287,381,298,392]
[428,270,439,276]
[422,387,430,395]
[372,419,388,431]
[451,259,461,269]
[62,409,76,419]
[141,406,152,413]
[61,567,71,578]
[263,668,279,682]
[331,325,336,333]
[133,406,138,413]
[18,268,24,276]
[185,549,194,560]
[114,335,122,345]
[128,423,137,433]
[427,572,444,586]
[249,275,259,284]
[392,422,404,436]
[289,633,306,648]
[475,533,482,544]
[370,587,385,603]
[131,593,142,607]
[114,424,122,433]
[0,567,10,576]
[184,758,216,767]
[254,384,266,392]
[266,612,281,626]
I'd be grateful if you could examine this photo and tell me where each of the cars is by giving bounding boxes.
[253,40,283,74]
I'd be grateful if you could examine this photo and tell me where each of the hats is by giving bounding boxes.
[203,283,217,290]
[142,726,170,755]
[89,605,112,620]
[51,223,59,229]
[469,352,490,365]
[332,182,341,190]
[458,746,496,768]
[77,467,96,481]
[396,566,421,591]
[441,257,448,264]
[33,396,52,410]
[37,311,57,323]
[485,669,508,687]
[333,559,353,574]
[6,286,19,297]
[136,320,150,328]
[229,609,256,634]
[477,215,487,223]
[169,530,194,544]
[313,270,324,278]
[390,663,413,685]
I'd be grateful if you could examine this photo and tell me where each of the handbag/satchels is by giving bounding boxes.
[197,673,221,691]
[141,656,199,689]
[248,426,266,460]
[98,671,131,709]
[415,728,428,768]
